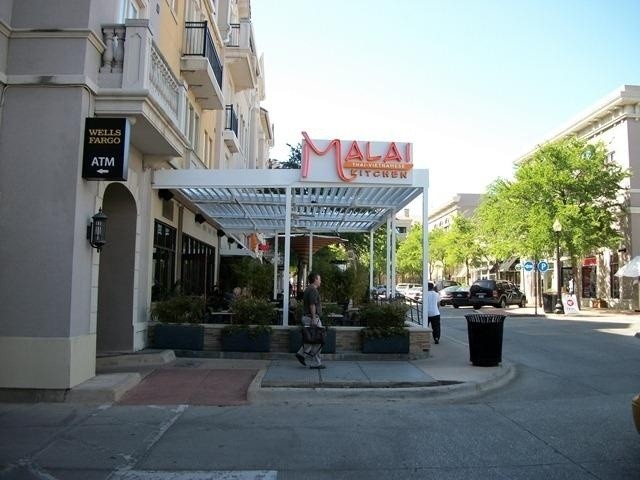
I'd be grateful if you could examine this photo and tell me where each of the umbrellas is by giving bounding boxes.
[614,256,640,278]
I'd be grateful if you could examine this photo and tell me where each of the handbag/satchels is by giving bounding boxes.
[302,325,326,344]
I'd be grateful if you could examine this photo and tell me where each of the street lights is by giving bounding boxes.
[554,221,565,314]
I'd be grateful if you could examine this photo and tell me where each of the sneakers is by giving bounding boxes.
[295,353,307,366]
[310,365,326,369]
[435,337,439,344]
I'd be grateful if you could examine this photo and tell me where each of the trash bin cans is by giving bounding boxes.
[465,313,507,366]
[543,292,552,313]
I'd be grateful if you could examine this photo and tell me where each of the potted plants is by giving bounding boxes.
[221,293,278,352]
[148,296,204,351]
[290,298,337,353]
[359,296,409,353]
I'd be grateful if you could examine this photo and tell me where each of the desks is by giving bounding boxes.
[212,301,283,324]
[320,302,359,326]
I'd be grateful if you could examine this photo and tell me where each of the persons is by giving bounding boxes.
[294,272,326,369]
[421,282,440,344]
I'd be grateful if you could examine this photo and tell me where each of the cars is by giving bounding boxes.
[371,279,528,310]
